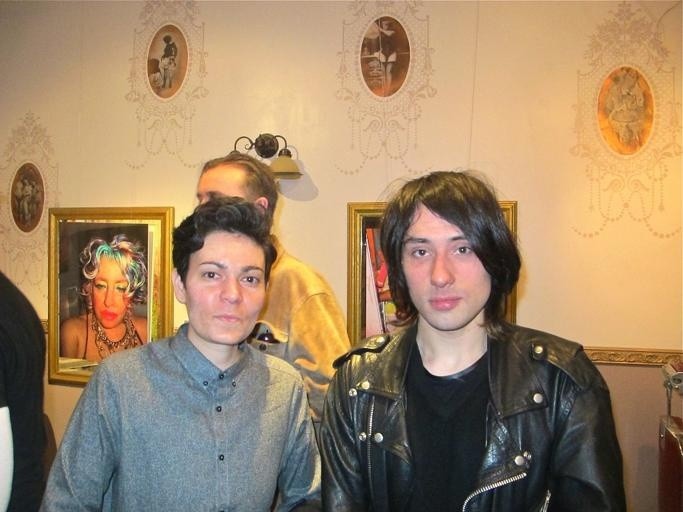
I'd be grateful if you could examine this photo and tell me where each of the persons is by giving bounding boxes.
[59,234,147,363]
[196,150,351,451]
[0,267,46,511]
[18,177,38,224]
[374,18,398,94]
[158,36,177,89]
[37,196,323,511]
[604,68,647,145]
[316,170,628,510]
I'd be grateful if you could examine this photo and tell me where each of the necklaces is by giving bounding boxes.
[91,313,137,359]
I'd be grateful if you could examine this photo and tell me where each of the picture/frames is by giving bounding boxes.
[46,206,174,387]
[346,200,518,349]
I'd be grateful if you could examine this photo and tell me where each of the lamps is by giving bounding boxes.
[232,132,303,175]
